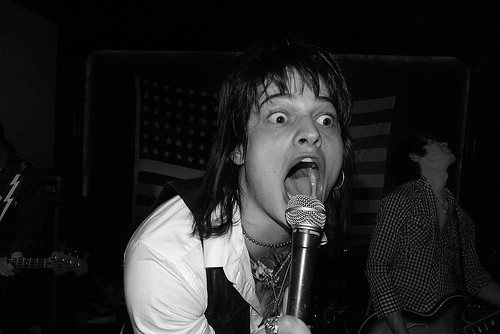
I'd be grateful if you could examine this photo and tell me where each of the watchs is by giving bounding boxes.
[264,316,279,334]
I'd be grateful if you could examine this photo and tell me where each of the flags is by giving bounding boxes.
[130,72,400,254]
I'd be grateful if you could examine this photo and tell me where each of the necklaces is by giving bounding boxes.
[241,226,294,318]
[434,195,448,214]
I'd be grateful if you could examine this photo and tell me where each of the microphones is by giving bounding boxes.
[285,195,327,323]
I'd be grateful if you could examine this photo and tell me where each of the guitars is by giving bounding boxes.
[0,249,89,277]
[356,293,500,334]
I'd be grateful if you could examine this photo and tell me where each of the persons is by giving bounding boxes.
[366,125,500,334]
[0,129,51,334]
[121,38,353,334]
